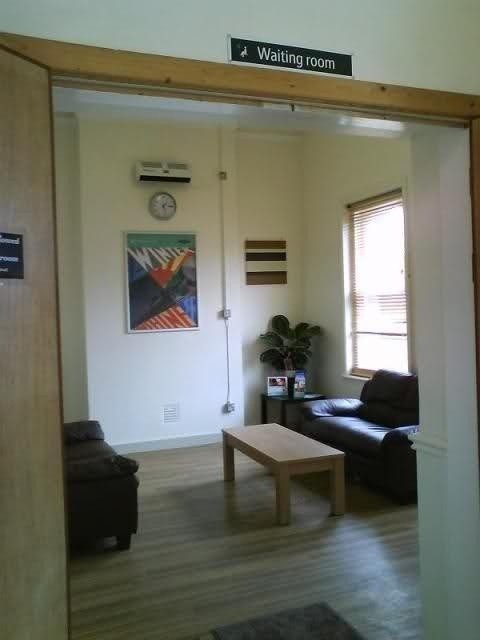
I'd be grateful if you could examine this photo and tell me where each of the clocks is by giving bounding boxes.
[149,192,177,221]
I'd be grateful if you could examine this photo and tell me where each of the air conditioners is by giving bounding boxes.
[135,160,193,184]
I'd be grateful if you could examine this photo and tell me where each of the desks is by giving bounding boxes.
[221,422,345,527]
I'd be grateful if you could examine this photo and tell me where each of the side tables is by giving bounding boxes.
[261,392,325,434]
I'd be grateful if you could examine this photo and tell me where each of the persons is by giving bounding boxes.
[278,379,285,386]
[269,379,277,386]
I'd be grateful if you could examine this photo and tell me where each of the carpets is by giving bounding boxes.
[210,601,366,640]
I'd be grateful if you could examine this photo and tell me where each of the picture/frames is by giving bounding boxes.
[123,231,199,333]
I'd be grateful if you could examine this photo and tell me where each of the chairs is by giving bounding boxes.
[297,369,419,506]
[62,419,138,550]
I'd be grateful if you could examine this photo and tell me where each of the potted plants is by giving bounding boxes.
[258,313,321,396]
